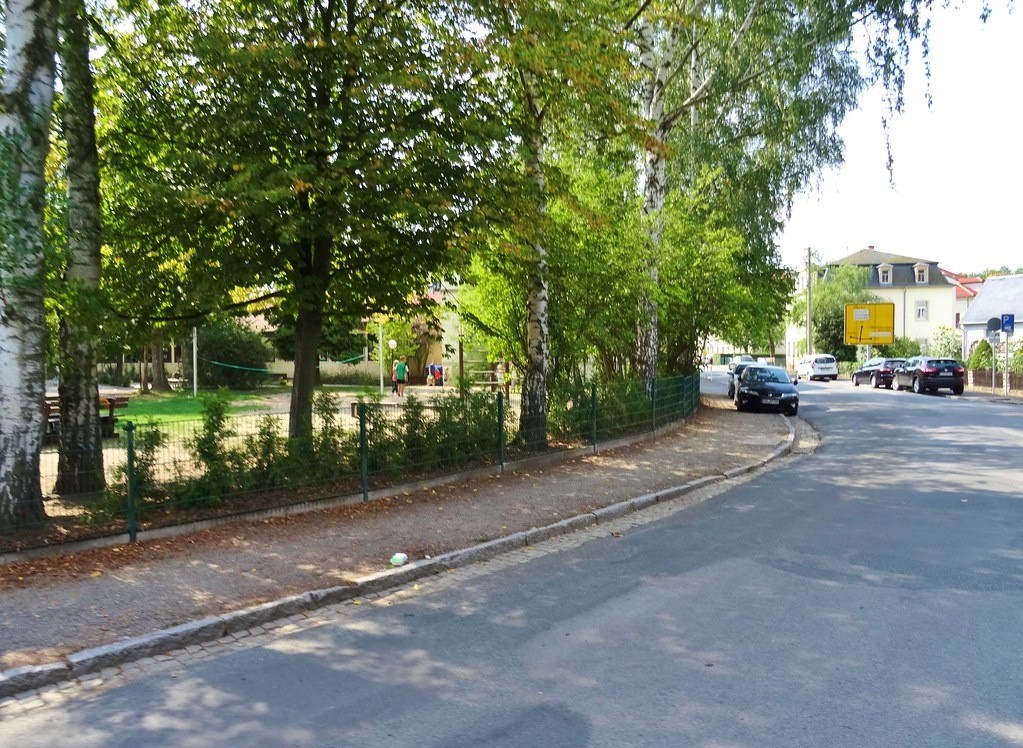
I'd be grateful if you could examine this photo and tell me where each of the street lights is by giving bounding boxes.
[389,340,397,375]
[373,320,383,395]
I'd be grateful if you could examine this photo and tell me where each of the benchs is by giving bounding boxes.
[38,395,130,437]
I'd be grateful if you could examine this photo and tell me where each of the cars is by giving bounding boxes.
[736,364,800,417]
[726,361,766,399]
[852,357,907,388]
[729,356,752,369]
[703,354,712,367]
[795,354,838,381]
[892,356,964,395]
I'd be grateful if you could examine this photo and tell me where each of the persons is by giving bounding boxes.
[396,356,408,397]
[392,359,400,396]
[429,358,435,387]
[494,357,517,392]
